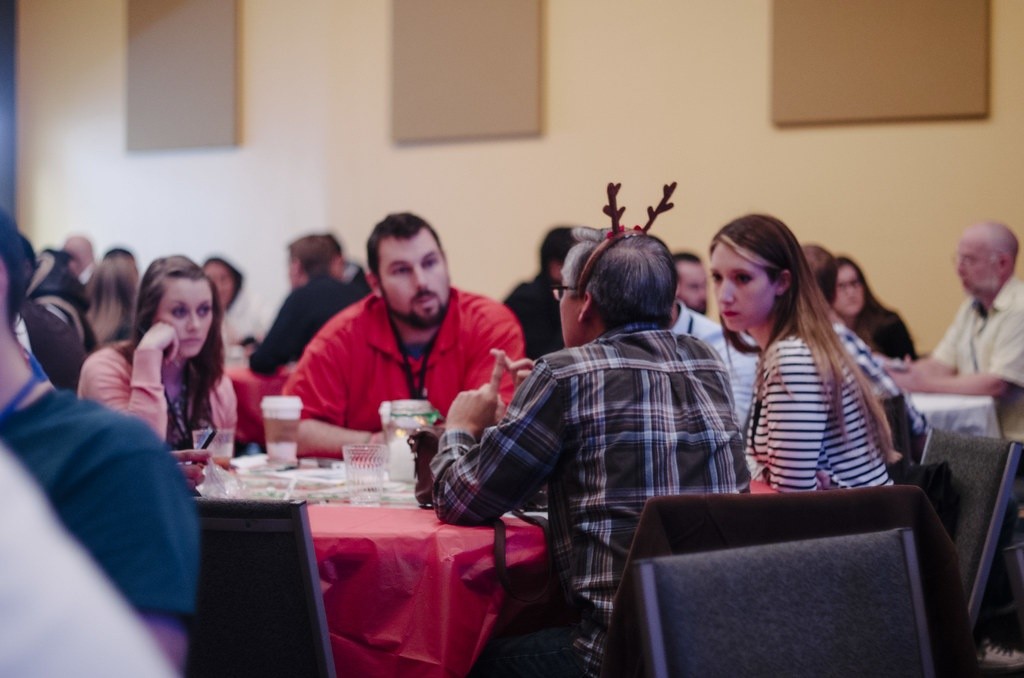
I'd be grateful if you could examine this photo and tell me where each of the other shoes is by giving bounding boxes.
[974,629,1024,675]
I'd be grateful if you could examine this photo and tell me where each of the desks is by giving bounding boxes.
[909,396,1002,439]
[223,362,299,441]
[306,503,552,678]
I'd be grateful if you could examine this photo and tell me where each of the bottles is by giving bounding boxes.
[381,399,440,483]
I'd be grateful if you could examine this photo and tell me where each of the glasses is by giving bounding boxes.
[551,281,577,301]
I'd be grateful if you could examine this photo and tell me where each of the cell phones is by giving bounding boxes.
[189,426,215,465]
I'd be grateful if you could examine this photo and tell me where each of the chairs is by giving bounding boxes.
[183,493,338,678]
[919,423,1022,632]
[630,524,938,678]
[597,484,973,678]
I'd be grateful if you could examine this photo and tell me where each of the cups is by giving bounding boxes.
[260,396,305,459]
[342,443,389,506]
[192,429,236,473]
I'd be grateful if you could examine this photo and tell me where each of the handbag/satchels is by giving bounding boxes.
[405,427,549,604]
[887,460,953,542]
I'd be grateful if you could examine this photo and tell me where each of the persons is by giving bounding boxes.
[883,222,1024,448]
[0,182,921,678]
